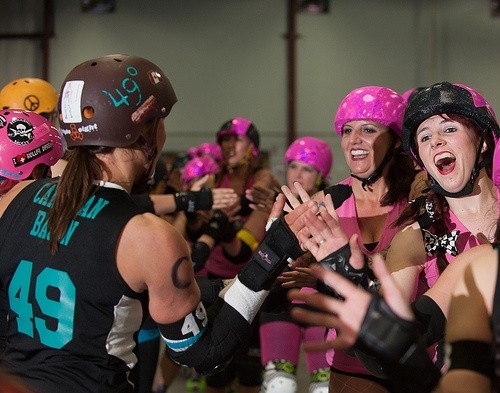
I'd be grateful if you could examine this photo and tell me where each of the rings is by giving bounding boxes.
[318,239,326,245]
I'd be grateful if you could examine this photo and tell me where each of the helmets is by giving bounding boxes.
[334,86,405,143]
[183,157,221,182]
[0,108,64,181]
[58,53,177,148]
[0,77,61,117]
[284,135,332,179]
[401,82,500,174]
[216,118,260,156]
[187,142,222,163]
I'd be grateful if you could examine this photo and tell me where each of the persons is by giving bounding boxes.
[287,134,499,393]
[296,82,500,368]
[258,130,338,393]
[139,116,283,392]
[1,51,327,392]
[2,75,60,127]
[441,245,500,393]
[0,108,240,216]
[276,84,428,393]
